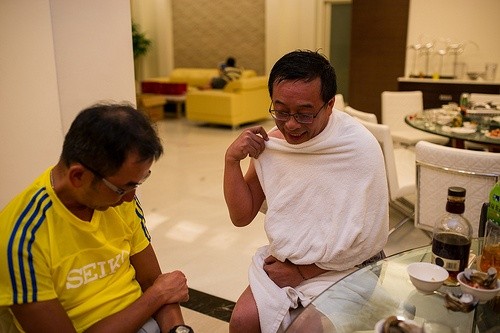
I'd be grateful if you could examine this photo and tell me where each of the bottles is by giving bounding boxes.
[487,180,500,226]
[430,187,473,287]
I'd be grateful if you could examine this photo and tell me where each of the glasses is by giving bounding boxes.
[81,161,152,195]
[269,100,329,123]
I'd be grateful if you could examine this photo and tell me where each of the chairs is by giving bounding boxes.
[330,91,500,239]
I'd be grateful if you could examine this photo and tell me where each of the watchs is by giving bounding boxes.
[168,325,194,333]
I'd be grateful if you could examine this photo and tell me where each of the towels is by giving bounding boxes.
[246,105,390,333]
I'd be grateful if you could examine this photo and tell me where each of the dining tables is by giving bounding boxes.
[282,235,500,333]
[405,111,500,152]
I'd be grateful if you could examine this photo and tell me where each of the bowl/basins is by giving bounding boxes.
[457,270,500,304]
[467,73,478,80]
[406,262,449,293]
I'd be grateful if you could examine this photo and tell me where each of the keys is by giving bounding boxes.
[434,290,479,313]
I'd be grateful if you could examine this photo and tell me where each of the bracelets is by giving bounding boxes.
[296,264,308,281]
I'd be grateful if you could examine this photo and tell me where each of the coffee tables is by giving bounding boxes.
[165,94,197,116]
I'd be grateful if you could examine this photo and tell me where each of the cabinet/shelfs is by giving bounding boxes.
[397,78,500,109]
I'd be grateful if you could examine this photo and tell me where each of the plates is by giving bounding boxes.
[485,133,500,139]
[452,127,476,134]
[374,315,424,333]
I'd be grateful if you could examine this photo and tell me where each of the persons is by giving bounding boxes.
[223,48,389,333]
[210,58,241,90]
[0,104,194,333]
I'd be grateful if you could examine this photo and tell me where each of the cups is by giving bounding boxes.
[480,221,500,272]
[470,237,484,256]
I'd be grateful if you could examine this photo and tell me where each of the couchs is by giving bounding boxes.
[136,68,273,130]
